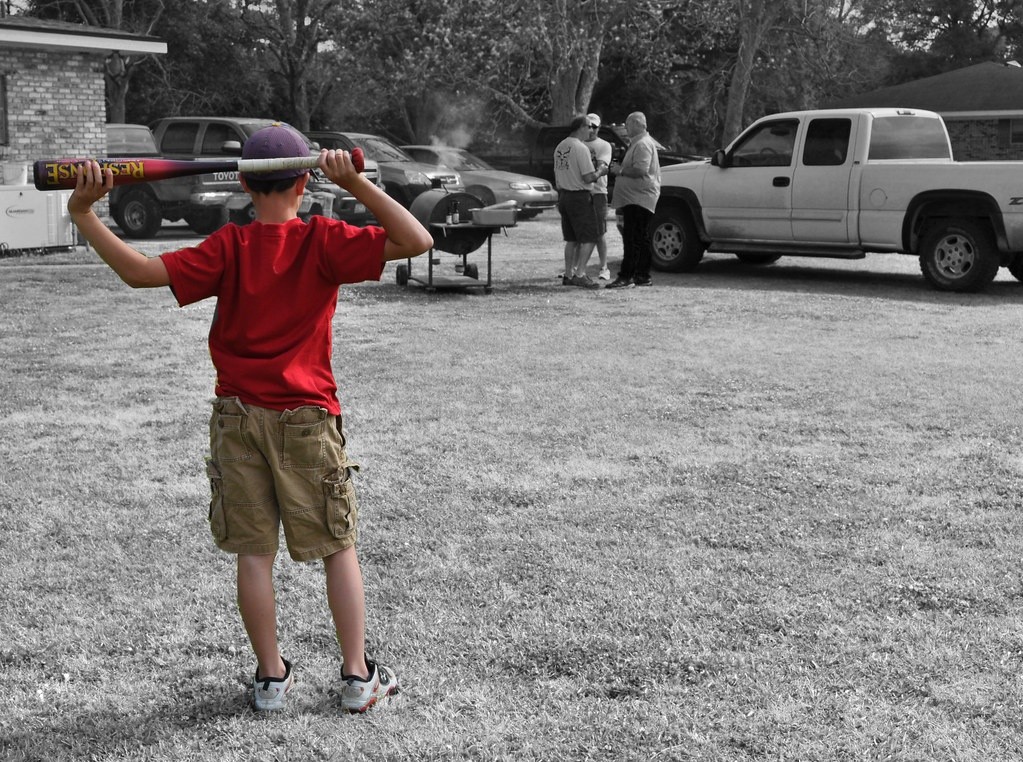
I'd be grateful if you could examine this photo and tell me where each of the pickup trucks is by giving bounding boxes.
[106,124,246,241]
[476,124,713,205]
[616,108,1023,295]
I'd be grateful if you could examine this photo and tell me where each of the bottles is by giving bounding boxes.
[610,158,619,174]
[453,202,460,224]
[445,205,453,225]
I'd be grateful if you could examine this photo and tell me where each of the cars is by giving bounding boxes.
[394,145,561,224]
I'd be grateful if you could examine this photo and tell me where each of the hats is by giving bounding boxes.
[241,121,319,181]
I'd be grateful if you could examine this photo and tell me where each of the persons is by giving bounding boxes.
[553,113,613,289]
[605,111,661,290]
[67,122,434,715]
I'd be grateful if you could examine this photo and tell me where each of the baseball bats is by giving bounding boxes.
[32,145,367,193]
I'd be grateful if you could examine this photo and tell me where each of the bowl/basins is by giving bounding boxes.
[468,207,514,225]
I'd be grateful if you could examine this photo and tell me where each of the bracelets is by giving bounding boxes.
[619,167,624,176]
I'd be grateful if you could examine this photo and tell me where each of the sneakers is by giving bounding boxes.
[604,277,635,289]
[598,269,610,280]
[621,278,652,289]
[562,274,573,285]
[340,652,398,714]
[254,655,294,711]
[571,273,600,289]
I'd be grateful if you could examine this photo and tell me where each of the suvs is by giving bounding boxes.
[302,130,466,211]
[140,117,386,228]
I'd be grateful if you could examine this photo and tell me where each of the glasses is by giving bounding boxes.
[584,125,591,129]
[592,123,600,129]
